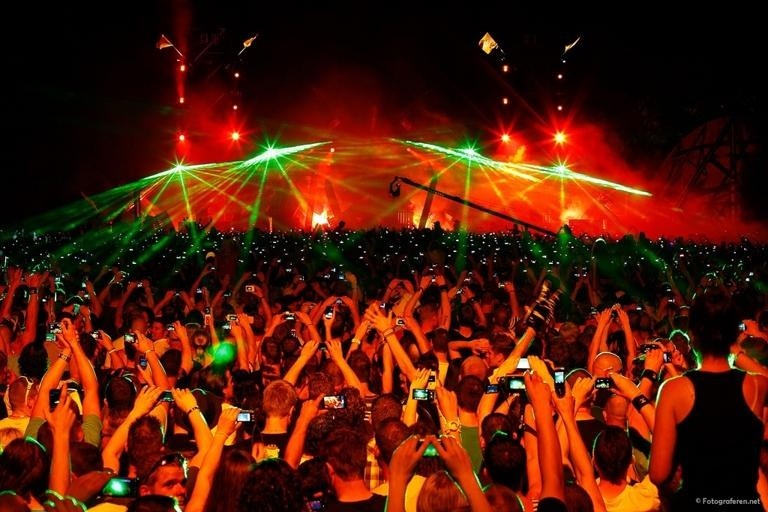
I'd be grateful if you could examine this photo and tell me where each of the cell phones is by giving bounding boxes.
[596,378,613,389]
[396,319,404,326]
[319,343,327,350]
[245,285,255,292]
[49,323,61,330]
[55,276,61,282]
[412,389,436,401]
[284,313,296,321]
[432,278,437,282]
[298,275,305,281]
[336,274,344,279]
[309,498,325,511]
[251,273,258,277]
[49,389,61,412]
[455,288,465,295]
[379,302,387,308]
[205,307,210,314]
[554,367,565,391]
[326,313,333,318]
[323,395,346,409]
[227,314,238,322]
[98,477,140,498]
[237,410,255,422]
[335,298,342,303]
[196,288,202,293]
[137,282,143,287]
[161,390,175,402]
[208,266,217,270]
[739,322,746,331]
[124,332,135,342]
[663,352,672,363]
[224,292,231,297]
[497,281,505,289]
[46,333,57,341]
[168,324,174,330]
[640,344,659,353]
[90,331,99,339]
[420,438,442,458]
[428,376,435,382]
[139,355,146,369]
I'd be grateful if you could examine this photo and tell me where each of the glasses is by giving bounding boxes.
[146,452,184,478]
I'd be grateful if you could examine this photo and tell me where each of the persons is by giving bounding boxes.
[0,221,767,512]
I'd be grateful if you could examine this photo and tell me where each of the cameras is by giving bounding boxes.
[485,376,526,394]
